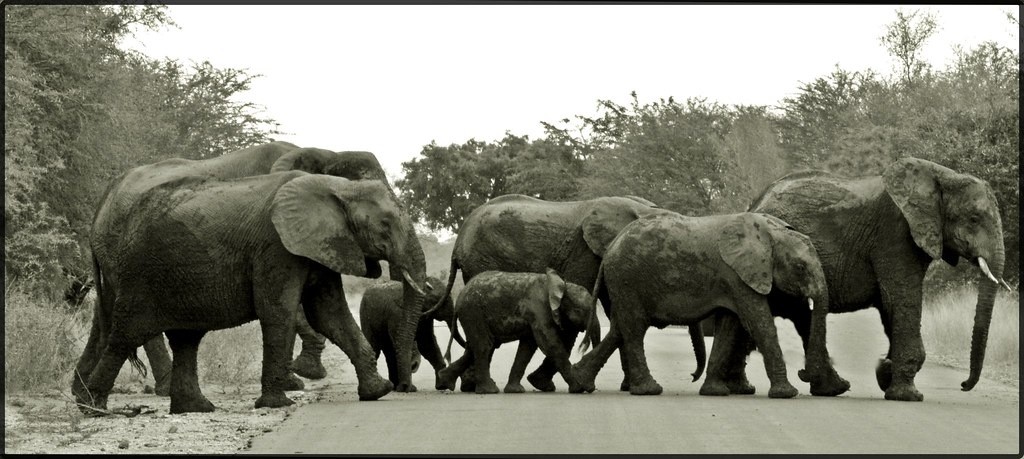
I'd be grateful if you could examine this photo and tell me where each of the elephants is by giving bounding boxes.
[72,142,1012,416]
